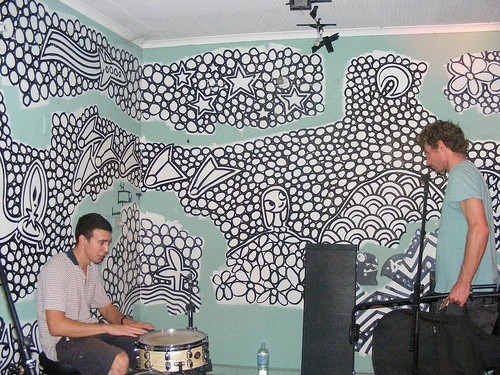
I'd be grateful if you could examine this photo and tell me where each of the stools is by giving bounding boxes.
[39,352,76,375]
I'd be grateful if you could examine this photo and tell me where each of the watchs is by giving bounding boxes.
[121,314,133,326]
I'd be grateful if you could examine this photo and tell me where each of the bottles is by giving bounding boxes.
[257,342,269,375]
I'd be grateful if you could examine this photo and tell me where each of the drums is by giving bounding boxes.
[133,328,212,374]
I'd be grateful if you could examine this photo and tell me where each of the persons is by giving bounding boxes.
[419,120,500,375]
[36,213,155,375]
